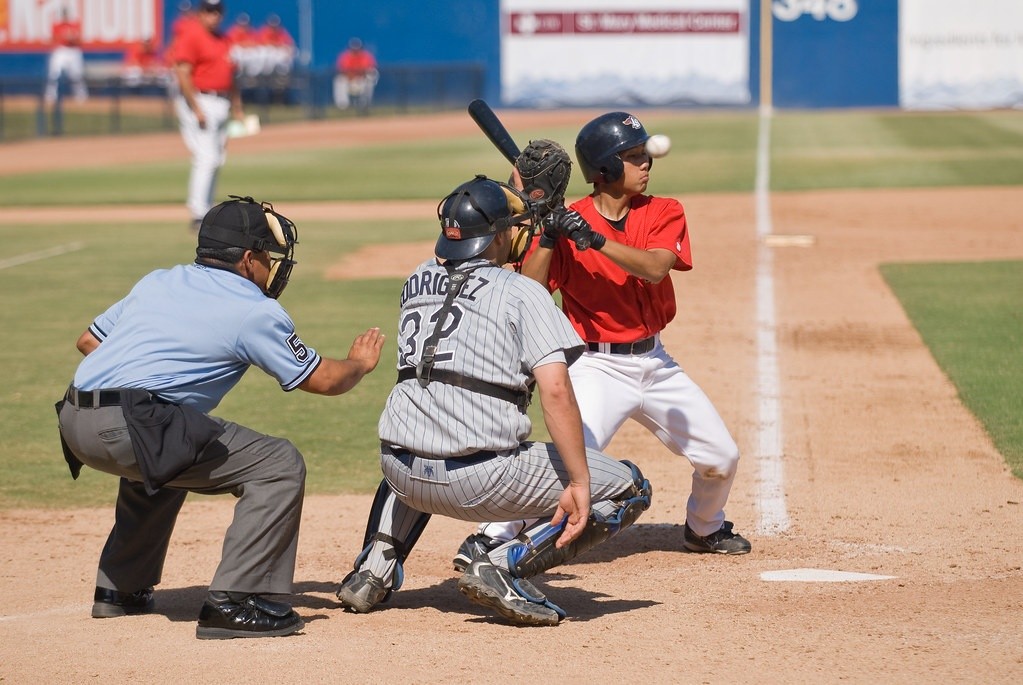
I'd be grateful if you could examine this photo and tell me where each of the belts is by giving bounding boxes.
[67,386,122,407]
[588,336,655,356]
[201,90,229,98]
[389,445,497,471]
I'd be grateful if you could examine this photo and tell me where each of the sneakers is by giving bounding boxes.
[683,520,750,555]
[458,553,560,626]
[453,534,490,570]
[338,569,389,613]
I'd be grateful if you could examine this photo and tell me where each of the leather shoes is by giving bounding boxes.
[195,596,306,639]
[91,587,156,617]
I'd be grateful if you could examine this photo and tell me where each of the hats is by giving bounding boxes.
[203,0,223,11]
[198,200,279,248]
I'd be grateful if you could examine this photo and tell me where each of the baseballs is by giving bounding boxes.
[648,134,671,159]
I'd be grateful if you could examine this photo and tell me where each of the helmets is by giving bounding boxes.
[434,178,509,260]
[575,112,653,183]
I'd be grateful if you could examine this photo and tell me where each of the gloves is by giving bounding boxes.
[555,210,598,247]
[543,205,566,240]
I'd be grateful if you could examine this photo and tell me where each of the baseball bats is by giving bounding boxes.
[467,99,591,252]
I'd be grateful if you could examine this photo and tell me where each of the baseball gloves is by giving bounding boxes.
[503,138,572,226]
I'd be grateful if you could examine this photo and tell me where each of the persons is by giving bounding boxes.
[338,174,650,625]
[57,195,386,637]
[452,112,751,572]
[331,41,380,112]
[176,0,245,230]
[123,4,299,96]
[42,7,89,108]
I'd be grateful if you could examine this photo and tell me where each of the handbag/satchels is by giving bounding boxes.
[120,388,228,497]
